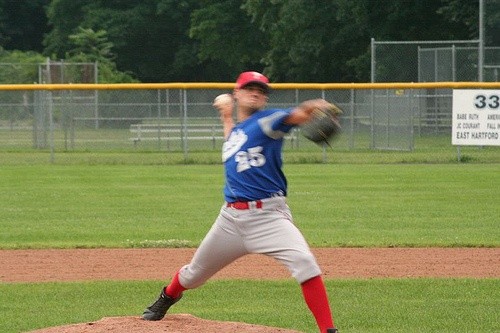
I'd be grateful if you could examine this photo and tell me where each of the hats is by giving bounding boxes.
[236,71,274,94]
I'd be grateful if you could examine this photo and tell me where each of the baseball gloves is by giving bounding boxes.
[300,100,344,146]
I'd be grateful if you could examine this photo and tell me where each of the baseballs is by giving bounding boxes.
[213,94,234,111]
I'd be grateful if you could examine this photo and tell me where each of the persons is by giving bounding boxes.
[141,71,338,333]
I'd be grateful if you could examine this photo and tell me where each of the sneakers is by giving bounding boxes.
[142,286,184,321]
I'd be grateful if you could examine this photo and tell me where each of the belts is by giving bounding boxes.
[227,200,262,209]
